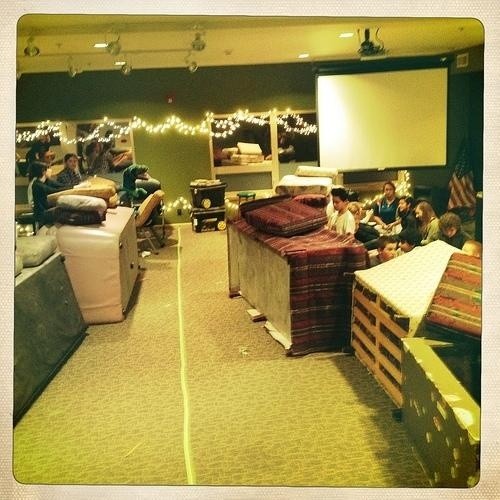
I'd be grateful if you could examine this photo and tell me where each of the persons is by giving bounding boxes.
[76,131,128,174]
[327,181,484,267]
[31,161,77,228]
[27,160,42,210]
[56,153,81,187]
[15,131,61,172]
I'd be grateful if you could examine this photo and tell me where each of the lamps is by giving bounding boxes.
[24,34,40,56]
[119,56,133,77]
[184,53,199,73]
[191,32,206,50]
[106,31,122,54]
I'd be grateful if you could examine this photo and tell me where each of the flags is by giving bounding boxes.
[447,142,477,216]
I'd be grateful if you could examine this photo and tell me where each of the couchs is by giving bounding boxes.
[221,141,265,166]
[225,168,484,487]
[16,163,166,434]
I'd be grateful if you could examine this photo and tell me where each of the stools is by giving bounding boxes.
[236,191,256,218]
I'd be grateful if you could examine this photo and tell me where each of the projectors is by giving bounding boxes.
[357,39,382,57]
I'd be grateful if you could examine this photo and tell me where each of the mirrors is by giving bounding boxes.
[16,118,136,186]
[208,110,318,175]
